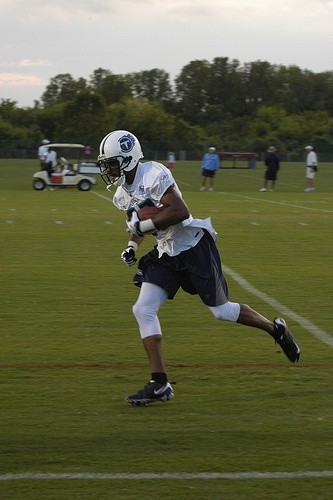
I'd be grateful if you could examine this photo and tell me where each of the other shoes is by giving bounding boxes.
[199,186,214,192]
[304,187,314,192]
[259,188,273,192]
[42,187,55,192]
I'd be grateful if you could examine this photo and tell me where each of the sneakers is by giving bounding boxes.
[272,316,300,364]
[127,378,174,406]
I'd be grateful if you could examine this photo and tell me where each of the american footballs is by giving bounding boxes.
[127,206,165,222]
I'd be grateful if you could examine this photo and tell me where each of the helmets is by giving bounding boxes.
[96,130,144,185]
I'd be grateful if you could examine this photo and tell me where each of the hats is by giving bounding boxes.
[267,146,277,152]
[208,147,216,151]
[40,139,50,144]
[304,146,313,149]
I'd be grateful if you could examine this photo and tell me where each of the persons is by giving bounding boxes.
[259,146,279,192]
[97,130,300,405]
[37,139,57,191]
[302,146,318,193]
[199,146,220,192]
[167,151,176,169]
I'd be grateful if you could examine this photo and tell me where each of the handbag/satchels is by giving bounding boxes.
[313,166,317,172]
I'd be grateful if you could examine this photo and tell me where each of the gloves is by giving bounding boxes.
[120,245,136,268]
[125,211,144,239]
